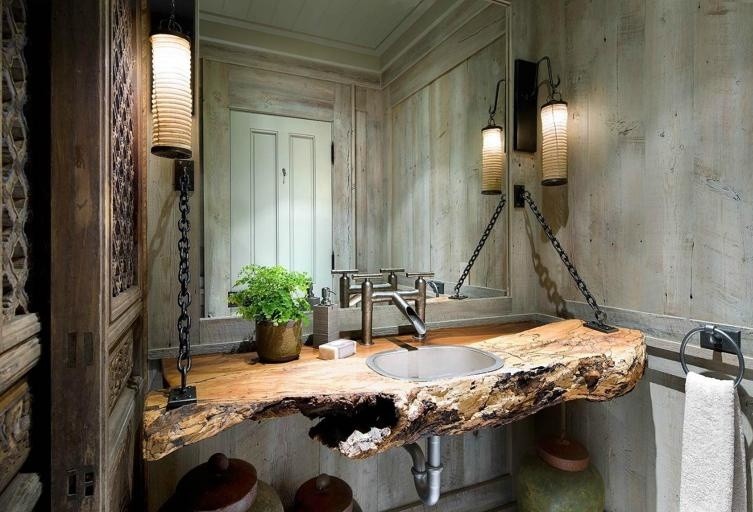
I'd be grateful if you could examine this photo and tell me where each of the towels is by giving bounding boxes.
[676,371,748,512]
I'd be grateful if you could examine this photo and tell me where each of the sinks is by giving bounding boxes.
[367,344,505,382]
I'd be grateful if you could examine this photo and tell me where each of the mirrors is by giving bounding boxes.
[196,0,514,320]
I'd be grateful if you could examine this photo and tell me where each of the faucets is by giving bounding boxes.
[351,271,435,345]
[330,267,405,309]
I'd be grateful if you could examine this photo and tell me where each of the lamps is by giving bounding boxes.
[148,3,196,161]
[480,76,507,196]
[514,54,570,188]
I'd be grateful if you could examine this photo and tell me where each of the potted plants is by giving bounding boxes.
[232,263,316,363]
